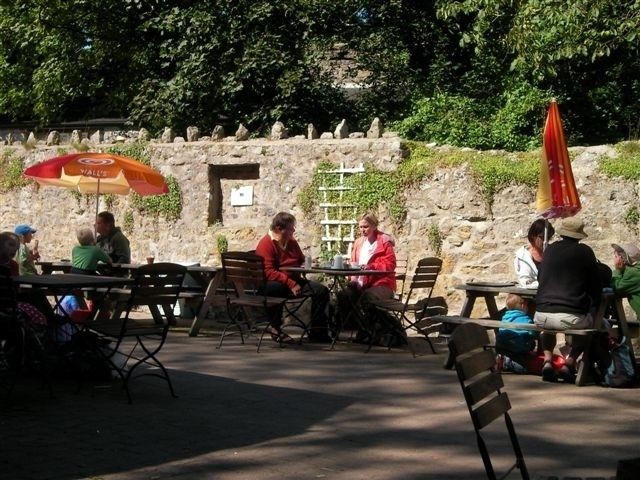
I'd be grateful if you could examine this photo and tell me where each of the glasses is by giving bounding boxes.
[536,235,550,242]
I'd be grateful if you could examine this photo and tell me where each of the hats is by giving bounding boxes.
[15,225,37,236]
[555,216,588,239]
[611,242,640,266]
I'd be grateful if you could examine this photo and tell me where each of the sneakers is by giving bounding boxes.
[495,353,504,374]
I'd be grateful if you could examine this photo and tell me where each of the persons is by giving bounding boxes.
[13,223,41,275]
[88,212,130,321]
[331,212,397,346]
[2,232,20,304]
[597,241,639,388]
[512,218,551,290]
[493,294,536,376]
[66,231,113,298]
[253,211,333,343]
[534,217,609,381]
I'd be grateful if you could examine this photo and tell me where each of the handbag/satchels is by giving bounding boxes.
[601,335,635,387]
[355,306,409,347]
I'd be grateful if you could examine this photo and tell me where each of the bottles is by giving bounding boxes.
[305,253,311,269]
[334,253,343,269]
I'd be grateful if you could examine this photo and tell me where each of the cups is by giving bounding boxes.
[147,257,154,264]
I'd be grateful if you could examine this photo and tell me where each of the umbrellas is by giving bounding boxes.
[537,98,581,254]
[22,153,167,242]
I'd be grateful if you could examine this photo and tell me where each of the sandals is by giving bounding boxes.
[541,360,558,383]
[270,332,294,343]
[559,363,575,384]
